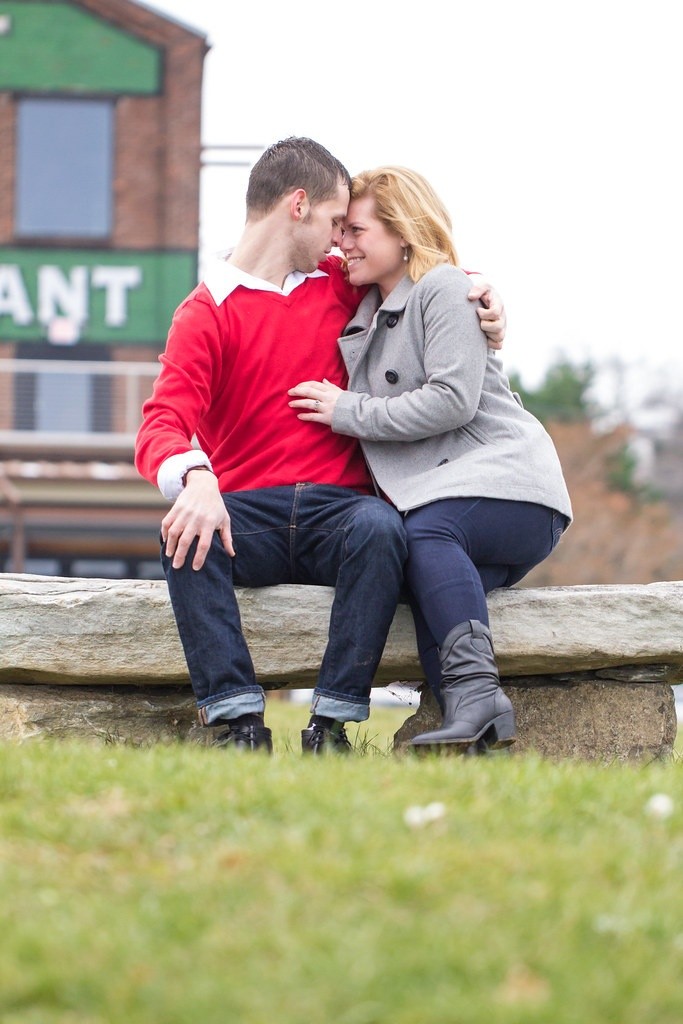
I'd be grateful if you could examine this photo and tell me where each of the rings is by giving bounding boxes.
[315,399,320,411]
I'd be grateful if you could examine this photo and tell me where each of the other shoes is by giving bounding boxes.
[302,727,352,756]
[219,726,271,753]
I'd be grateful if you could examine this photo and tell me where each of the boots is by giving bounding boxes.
[411,619,516,756]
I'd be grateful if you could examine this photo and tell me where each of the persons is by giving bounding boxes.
[288,166,573,754]
[133,138,505,759]
